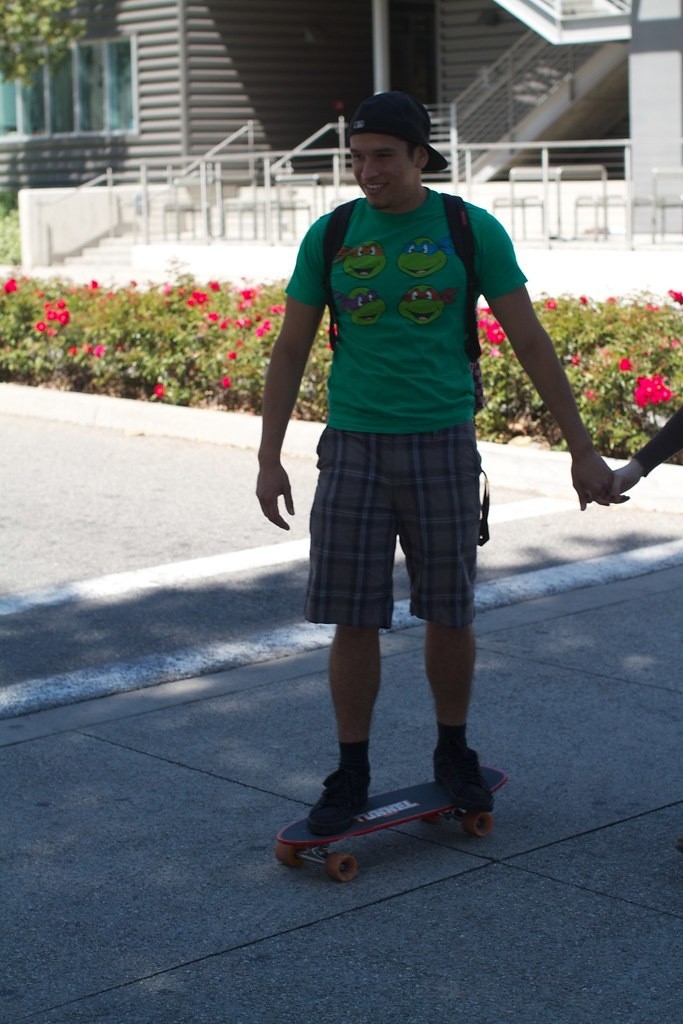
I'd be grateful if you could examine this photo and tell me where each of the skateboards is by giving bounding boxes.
[269,762,509,884]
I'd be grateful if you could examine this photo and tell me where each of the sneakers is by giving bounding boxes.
[307,768,371,835]
[433,746,494,812]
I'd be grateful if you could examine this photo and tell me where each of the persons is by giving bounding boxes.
[256,92,612,837]
[584,406,683,506]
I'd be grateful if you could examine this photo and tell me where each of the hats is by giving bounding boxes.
[347,91,448,173]
